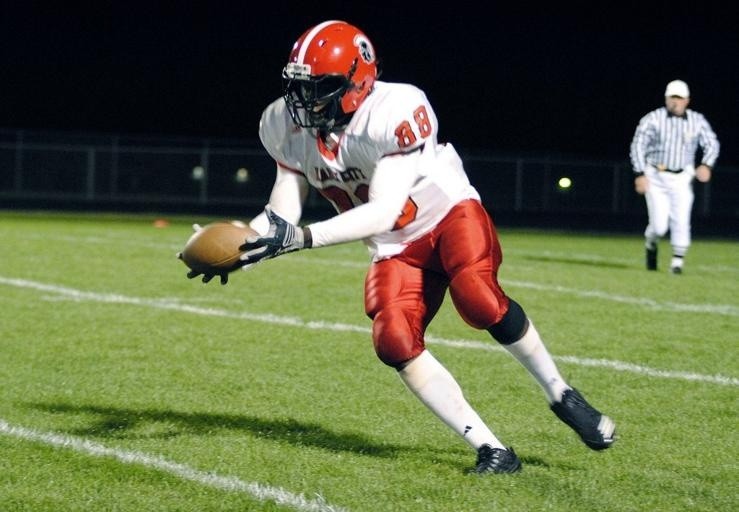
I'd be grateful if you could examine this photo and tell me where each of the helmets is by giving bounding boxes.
[278,21,378,131]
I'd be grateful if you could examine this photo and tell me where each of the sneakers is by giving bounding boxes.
[549,386,617,452]
[671,257,684,274]
[463,443,523,477]
[645,245,659,271]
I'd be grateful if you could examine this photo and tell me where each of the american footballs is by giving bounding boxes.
[182,223,260,275]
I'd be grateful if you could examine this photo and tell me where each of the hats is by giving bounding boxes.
[664,79,690,99]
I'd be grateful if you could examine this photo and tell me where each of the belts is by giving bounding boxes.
[652,164,683,175]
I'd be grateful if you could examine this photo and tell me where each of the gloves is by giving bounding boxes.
[238,204,312,272]
[175,222,228,285]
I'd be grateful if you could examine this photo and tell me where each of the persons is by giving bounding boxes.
[628,79,721,277]
[176,17,618,475]
